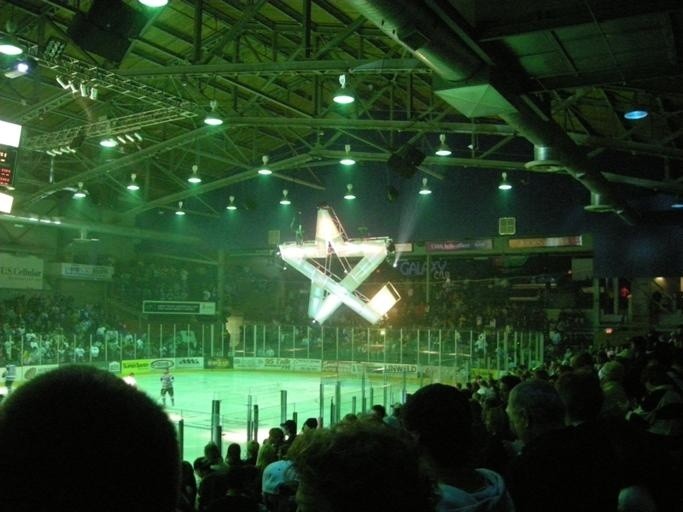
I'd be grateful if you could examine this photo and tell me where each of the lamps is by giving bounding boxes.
[1,24,23,56]
[333,78,355,104]
[257,155,273,175]
[343,184,356,200]
[435,134,453,156]
[72,187,88,199]
[56,70,100,104]
[123,173,142,192]
[2,57,38,80]
[188,164,201,183]
[622,92,649,123]
[339,143,356,166]
[418,179,432,195]
[278,189,291,205]
[175,201,186,216]
[226,196,237,211]
[203,100,223,126]
[498,172,512,190]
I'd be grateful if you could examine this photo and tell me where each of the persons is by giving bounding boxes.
[1,259,683,511]
